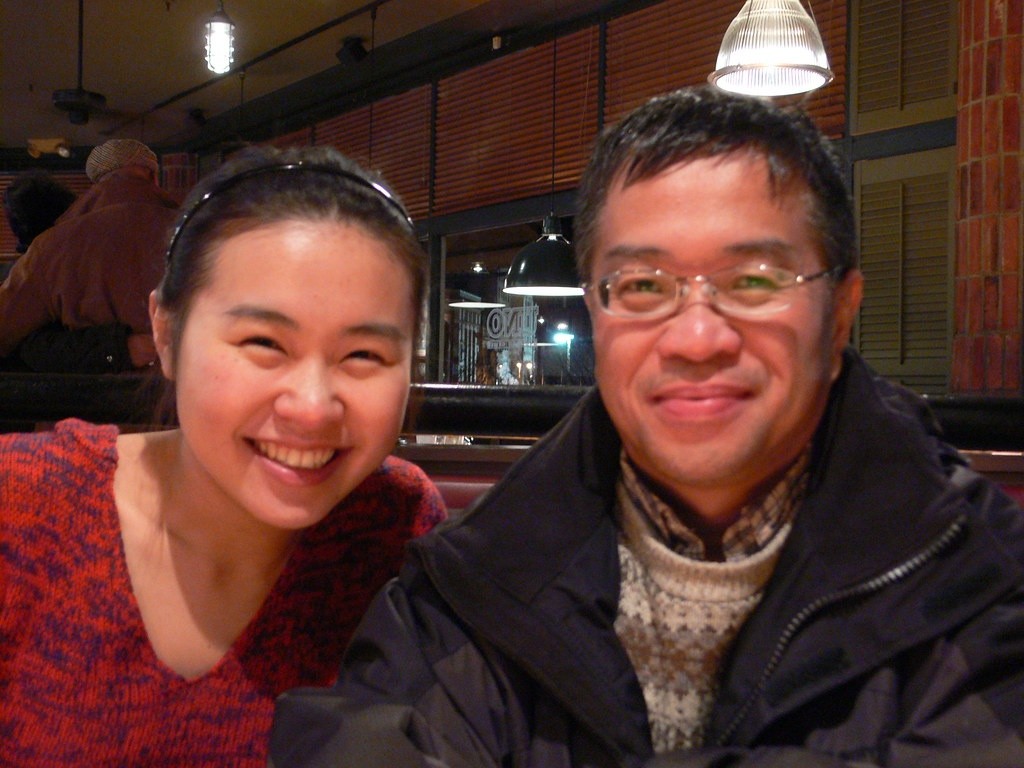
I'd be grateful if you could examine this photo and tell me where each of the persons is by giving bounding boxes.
[267,84,1024,768]
[1,138,448,768]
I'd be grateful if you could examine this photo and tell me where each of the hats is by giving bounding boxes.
[84,137,160,181]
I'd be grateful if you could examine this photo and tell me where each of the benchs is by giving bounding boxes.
[395,441,1023,528]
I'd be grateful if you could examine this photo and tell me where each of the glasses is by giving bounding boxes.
[583,260,827,321]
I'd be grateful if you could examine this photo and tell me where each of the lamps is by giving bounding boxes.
[705,0,836,101]
[200,0,237,76]
[501,1,585,300]
